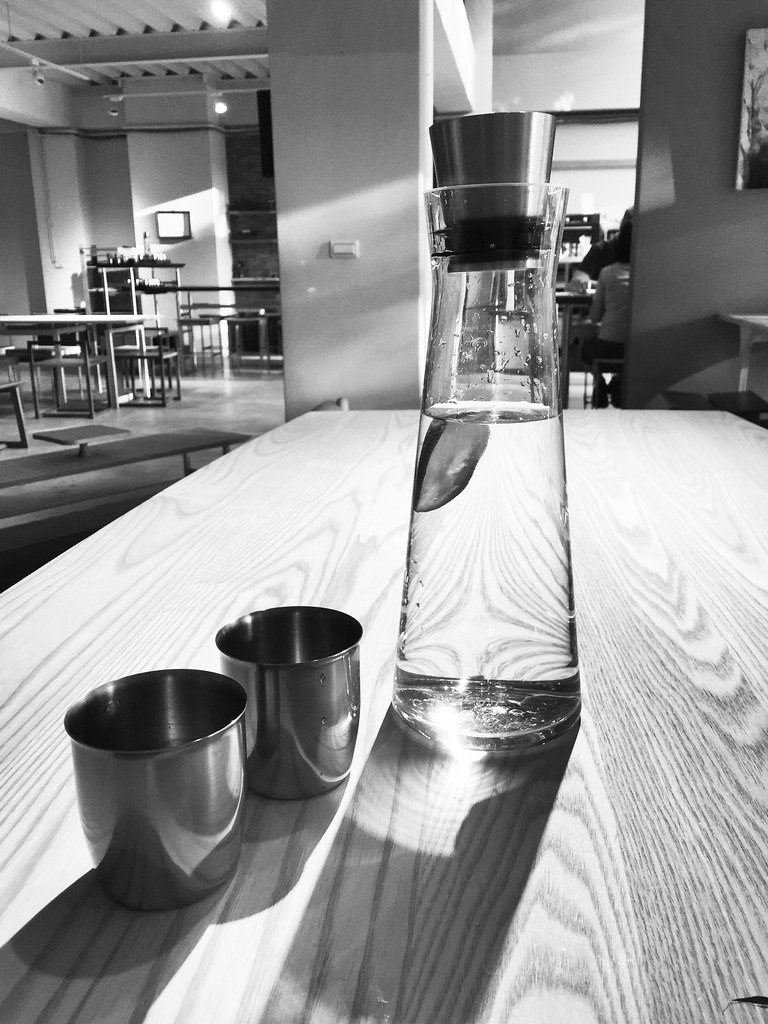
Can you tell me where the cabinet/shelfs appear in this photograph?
[225,207,281,290]
[85,244,190,378]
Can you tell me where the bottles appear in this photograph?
[390,108,583,753]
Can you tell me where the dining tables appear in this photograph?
[0,409,767,1024]
[166,284,283,372]
[554,289,596,409]
[0,313,163,410]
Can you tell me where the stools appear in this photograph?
[6,330,84,404]
[582,359,622,407]
[0,381,31,450]
[555,213,601,293]
[0,345,26,398]
[113,329,182,409]
[26,341,108,419]
[178,304,222,362]
[708,390,768,428]
[225,309,274,371]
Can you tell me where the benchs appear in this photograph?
[0,428,252,553]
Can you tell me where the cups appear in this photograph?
[214,604,363,801]
[63,668,251,913]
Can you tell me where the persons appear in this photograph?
[579,219,633,408]
[571,206,635,290]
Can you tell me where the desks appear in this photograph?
[716,311,768,396]
